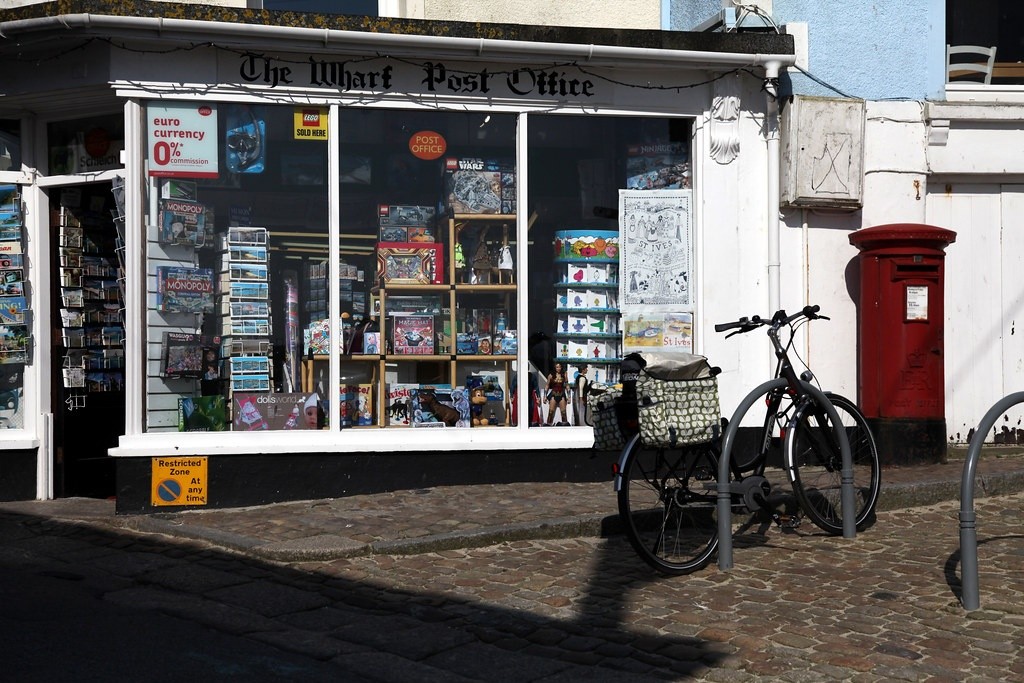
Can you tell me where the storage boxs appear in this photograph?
[158,150,519,428]
[627,130,692,189]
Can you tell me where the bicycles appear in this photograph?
[609,302,884,577]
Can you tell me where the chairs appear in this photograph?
[945,43,997,86]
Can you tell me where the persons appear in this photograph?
[495,312,508,334]
[510,362,541,425]
[292,403,300,428]
[542,362,571,427]
[304,393,320,429]
[574,364,589,426]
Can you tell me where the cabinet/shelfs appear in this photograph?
[213,229,276,393]
[58,185,127,411]
[551,230,621,392]
[303,207,518,427]
[0,181,32,365]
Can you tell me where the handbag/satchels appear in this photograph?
[624,350,723,446]
[587,380,625,450]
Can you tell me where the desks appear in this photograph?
[949,62,1024,84]
